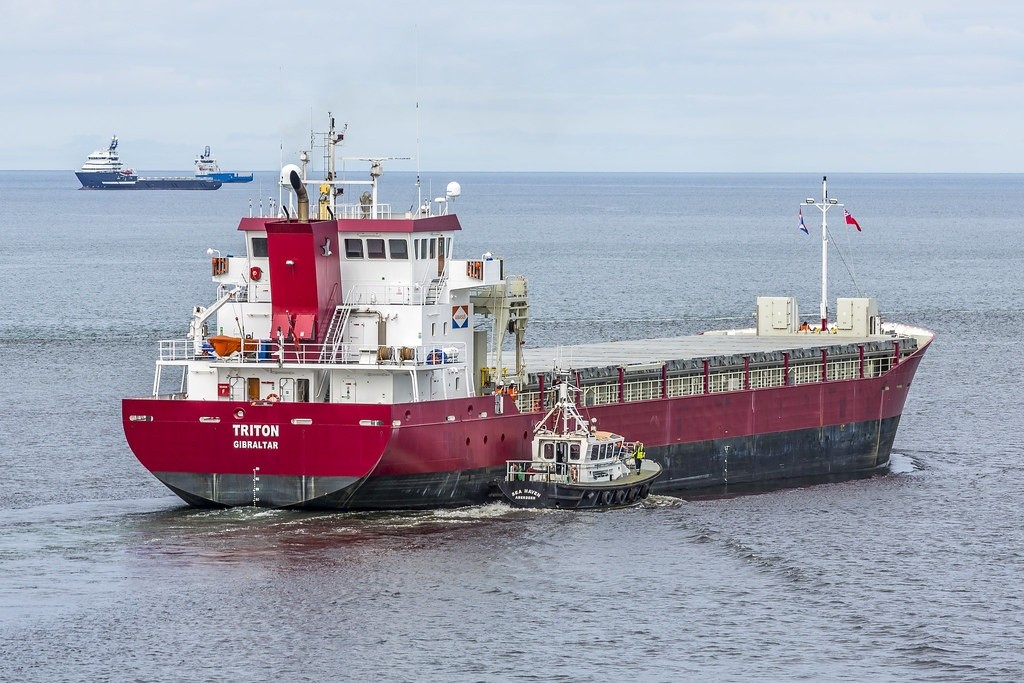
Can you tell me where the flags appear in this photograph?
[797,208,810,235]
[844,209,863,232]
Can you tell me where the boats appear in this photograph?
[117,109,936,511]
[193,145,254,183]
[493,341,663,510]
[74,135,223,190]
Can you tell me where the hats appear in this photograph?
[511,380,515,383]
[500,381,504,385]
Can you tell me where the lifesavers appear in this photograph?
[629,488,638,501]
[602,491,611,505]
[251,267,261,281]
[616,490,625,503]
[266,394,280,403]
[571,467,578,481]
[468,263,481,278]
[641,487,649,499]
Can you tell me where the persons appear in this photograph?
[630,440,646,475]
[800,322,812,331]
[552,376,562,403]
[496,381,506,396]
[508,380,518,401]
